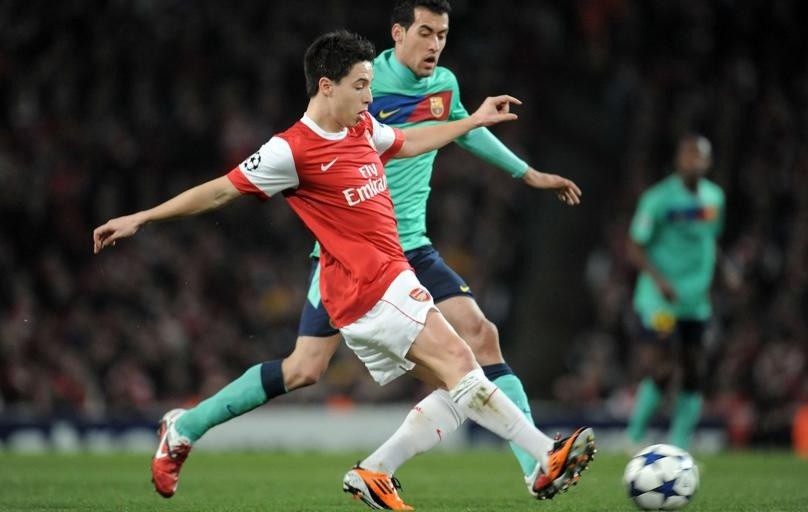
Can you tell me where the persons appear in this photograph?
[621,133,748,457]
[89,29,599,511]
[147,0,585,502]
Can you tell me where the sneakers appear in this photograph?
[343,461,415,512]
[151,409,191,497]
[524,425,597,500]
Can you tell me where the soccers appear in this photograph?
[625,444,700,511]
